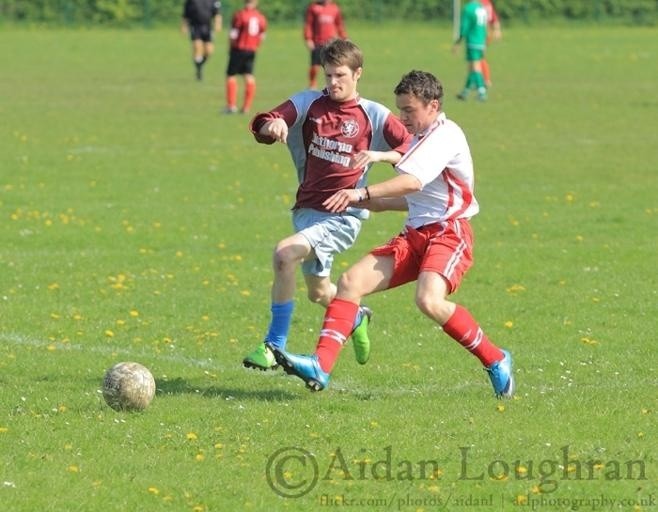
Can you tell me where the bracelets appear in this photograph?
[365,187,370,200]
[356,188,363,202]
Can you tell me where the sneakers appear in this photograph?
[274,349,330,391]
[243,343,280,369]
[484,350,514,400]
[351,307,371,364]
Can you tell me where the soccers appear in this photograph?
[103,362,155,411]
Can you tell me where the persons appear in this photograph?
[304,0,351,90]
[455,0,488,101]
[274,70,514,399]
[222,0,268,114]
[182,0,222,80]
[243,39,414,371]
[478,0,501,87]
